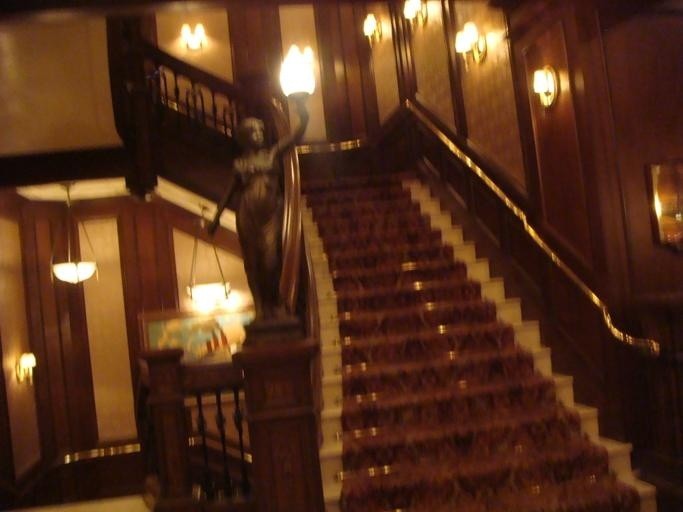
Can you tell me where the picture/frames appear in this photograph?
[140,305,257,368]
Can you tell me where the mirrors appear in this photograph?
[643,159,683,254]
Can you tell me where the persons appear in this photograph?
[206,95,307,322]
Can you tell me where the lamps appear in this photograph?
[15,351,38,386]
[363,13,382,49]
[403,0,428,33]
[533,64,558,111]
[179,1,210,52]
[183,201,253,325]
[48,180,100,284]
[454,20,488,73]
[279,44,316,97]
